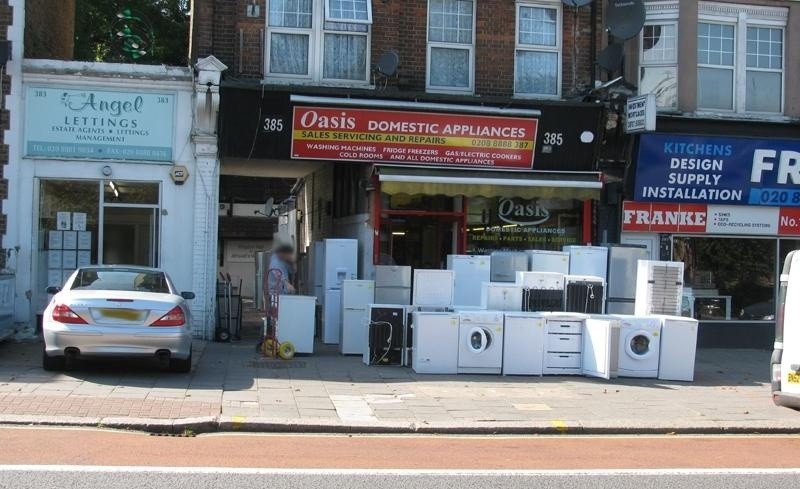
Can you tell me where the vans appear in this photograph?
[769,248,800,416]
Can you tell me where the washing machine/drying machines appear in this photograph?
[459,310,504,374]
[615,313,662,378]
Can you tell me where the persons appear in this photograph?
[257,245,297,350]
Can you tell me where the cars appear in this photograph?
[39,262,195,375]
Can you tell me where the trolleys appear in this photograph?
[256,268,295,361]
[215,270,240,344]
